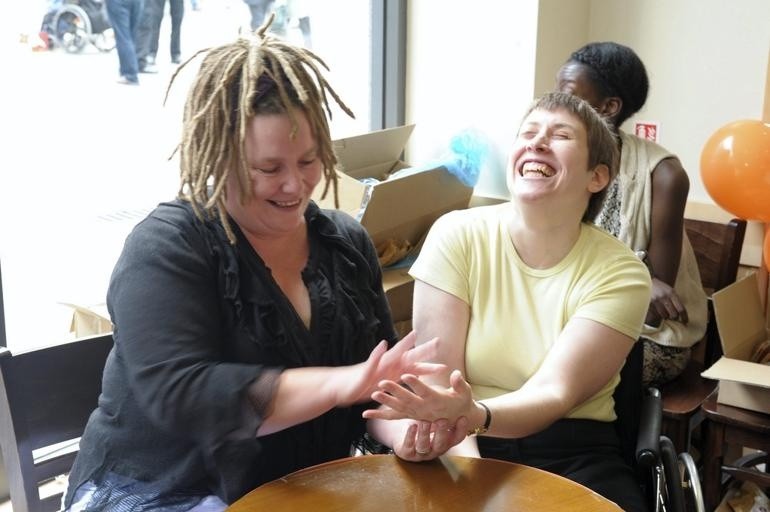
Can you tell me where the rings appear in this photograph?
[416,448,432,456]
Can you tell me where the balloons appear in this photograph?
[700,118,770,273]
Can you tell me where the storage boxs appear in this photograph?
[700,275,769,416]
[380,262,417,322]
[310,125,473,263]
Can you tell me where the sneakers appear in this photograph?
[116,57,183,87]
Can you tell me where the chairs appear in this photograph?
[692,385,769,512]
[1,336,116,512]
[644,218,748,476]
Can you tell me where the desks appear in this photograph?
[224,454,624,512]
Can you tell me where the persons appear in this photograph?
[555,41,708,387]
[362,91,652,512]
[105,0,184,84]
[64,11,448,512]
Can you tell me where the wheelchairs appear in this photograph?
[35,1,116,54]
[612,335,707,512]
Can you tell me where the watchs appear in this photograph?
[466,401,491,437]
